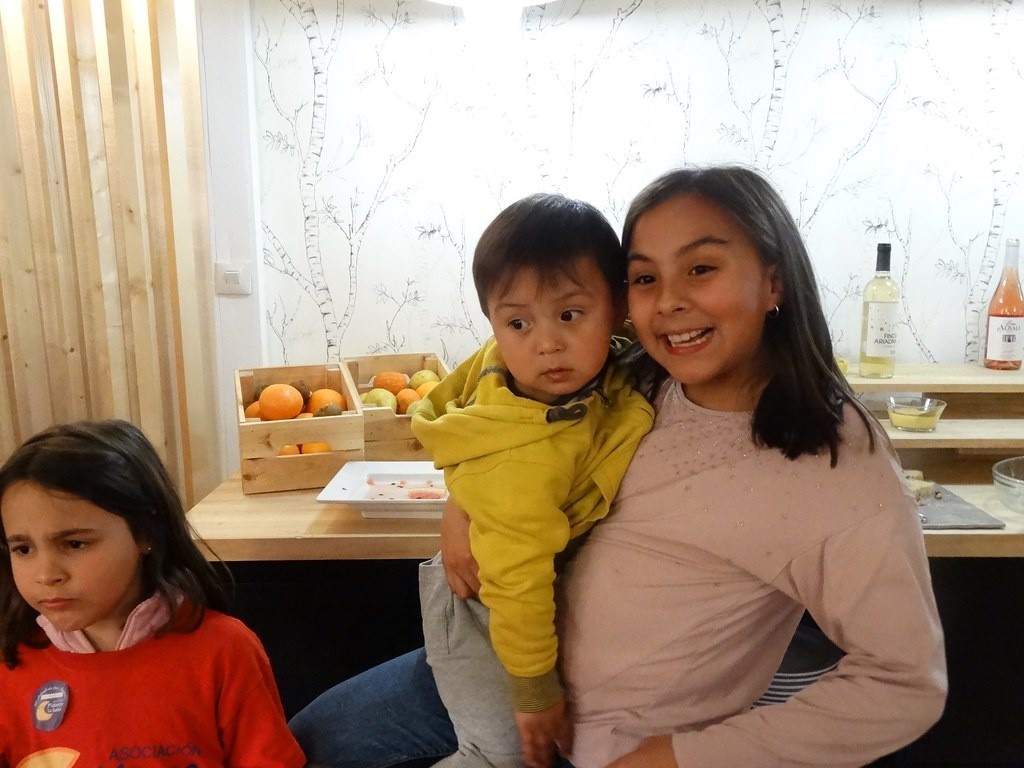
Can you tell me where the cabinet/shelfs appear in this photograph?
[839,363,1024,452]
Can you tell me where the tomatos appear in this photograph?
[243,383,347,456]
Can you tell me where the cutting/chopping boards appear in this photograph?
[917,479,1006,528]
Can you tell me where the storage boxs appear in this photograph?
[234,362,364,495]
[339,349,450,461]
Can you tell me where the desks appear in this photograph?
[184,463,1024,561]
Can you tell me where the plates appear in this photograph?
[317,461,448,519]
[750,625,843,709]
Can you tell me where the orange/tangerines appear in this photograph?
[395,388,423,415]
[372,371,407,394]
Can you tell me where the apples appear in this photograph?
[360,369,444,415]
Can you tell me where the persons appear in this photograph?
[285,166,947,768]
[0,418,309,768]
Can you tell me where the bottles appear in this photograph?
[984,238,1024,370]
[859,243,900,378]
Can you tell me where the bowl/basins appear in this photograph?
[885,397,947,431]
[992,457,1024,515]
